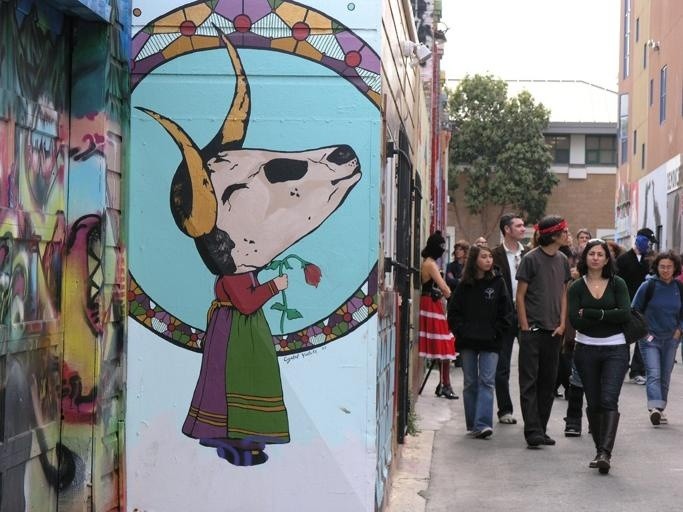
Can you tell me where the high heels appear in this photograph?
[435,383,460,399]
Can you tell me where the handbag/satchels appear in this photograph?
[618,308,649,345]
[431,269,445,302]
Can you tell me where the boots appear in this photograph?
[586,407,602,469]
[563,383,584,437]
[599,410,620,473]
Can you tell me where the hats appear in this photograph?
[636,226,660,244]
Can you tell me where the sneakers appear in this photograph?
[525,432,543,447]
[629,374,647,385]
[471,425,493,439]
[659,413,667,425]
[498,414,518,425]
[541,434,557,444]
[649,407,660,425]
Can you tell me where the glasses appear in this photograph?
[557,228,570,234]
[585,237,607,246]
